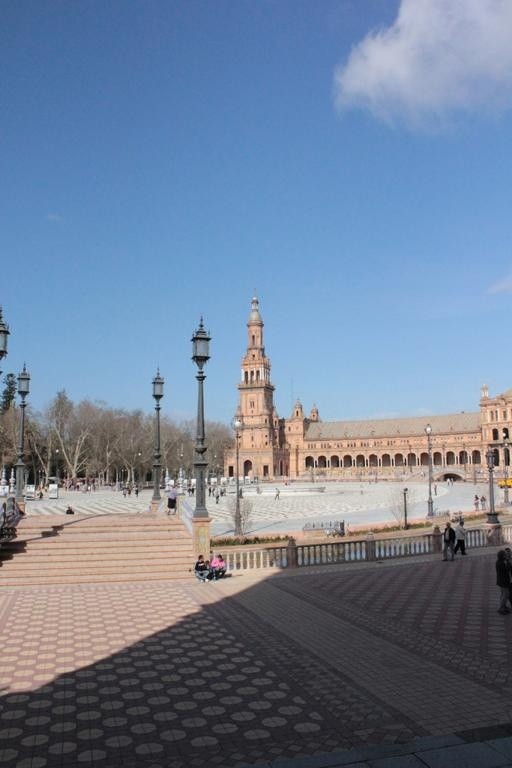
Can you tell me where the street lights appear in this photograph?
[55,448,60,481]
[406,440,478,485]
[230,416,244,537]
[191,313,213,517]
[402,487,410,528]
[12,360,31,499]
[486,444,501,523]
[150,364,166,500]
[0,304,12,363]
[502,472,510,502]
[424,422,436,516]
[503,440,510,473]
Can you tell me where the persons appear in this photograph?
[505,548,511,608]
[441,522,456,562]
[63,476,289,505]
[208,554,227,580]
[473,494,480,511]
[479,495,486,510]
[452,520,468,556]
[66,506,75,514]
[496,549,511,614]
[205,561,213,580]
[166,485,178,516]
[194,554,211,583]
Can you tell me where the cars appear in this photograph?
[324,528,344,538]
[497,478,512,488]
